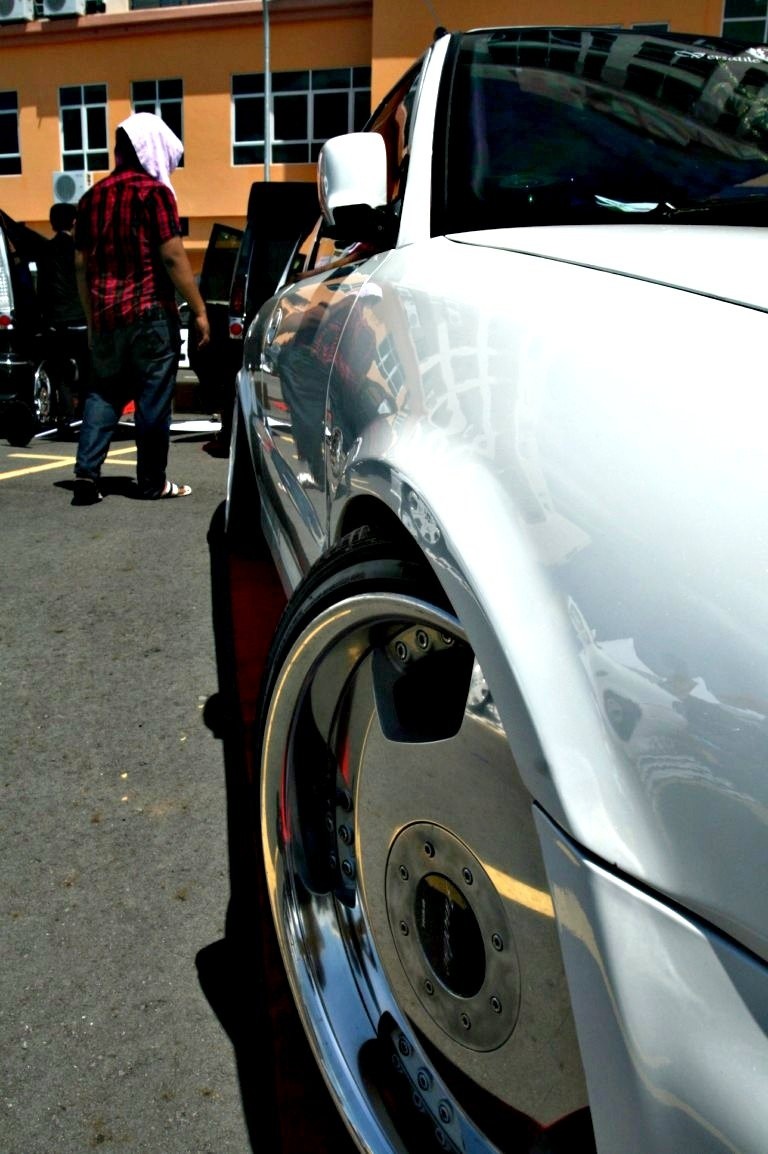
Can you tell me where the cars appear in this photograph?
[178,175,319,422]
[0,209,79,450]
[222,16,767,1152]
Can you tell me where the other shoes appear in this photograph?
[157,481,191,498]
[74,477,103,501]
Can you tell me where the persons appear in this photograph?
[72,112,209,502]
[270,282,383,492]
[49,203,76,251]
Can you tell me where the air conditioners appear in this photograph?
[0,0,34,22]
[53,170,94,205]
[42,0,86,17]
